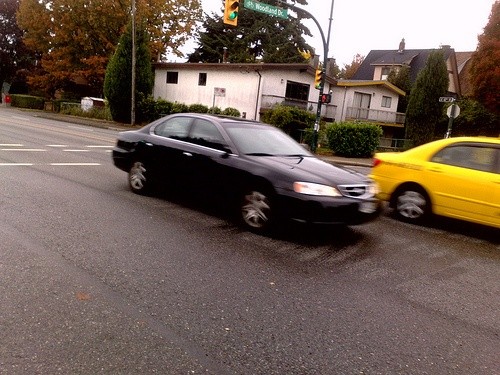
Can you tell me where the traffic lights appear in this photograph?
[224,0,239,26]
[315,70,323,90]
[322,94,332,104]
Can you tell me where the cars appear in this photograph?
[111,113,384,233]
[369,137,500,228]
